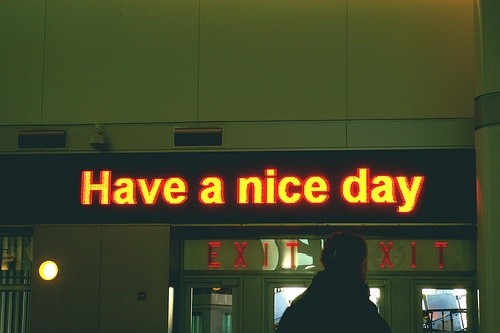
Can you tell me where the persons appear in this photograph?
[275,228,393,333]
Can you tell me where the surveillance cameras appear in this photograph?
[89,128,105,149]
